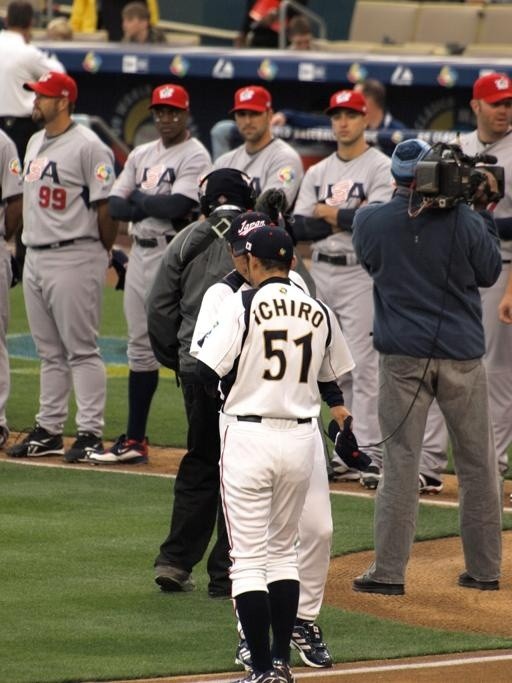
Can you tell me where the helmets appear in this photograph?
[197,167,256,216]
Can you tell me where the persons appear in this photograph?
[236,0,307,48]
[86,85,214,466]
[414,72,512,497]
[0,0,67,172]
[287,15,315,51]
[269,79,407,156]
[0,126,23,452]
[68,1,159,34]
[44,16,72,41]
[122,3,167,43]
[212,86,304,214]
[351,138,503,595]
[292,89,394,491]
[4,72,118,465]
[144,168,380,681]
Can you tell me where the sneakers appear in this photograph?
[84,434,151,465]
[5,422,66,457]
[62,429,106,463]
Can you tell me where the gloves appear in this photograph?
[323,415,372,474]
[113,245,128,289]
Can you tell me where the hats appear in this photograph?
[389,138,434,188]
[228,84,273,113]
[148,83,191,112]
[23,70,78,103]
[224,210,293,263]
[324,89,367,115]
[472,72,512,104]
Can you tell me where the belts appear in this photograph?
[235,414,314,424]
[32,239,74,250]
[317,252,347,266]
[136,234,174,247]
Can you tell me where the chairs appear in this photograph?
[348,0,512,58]
